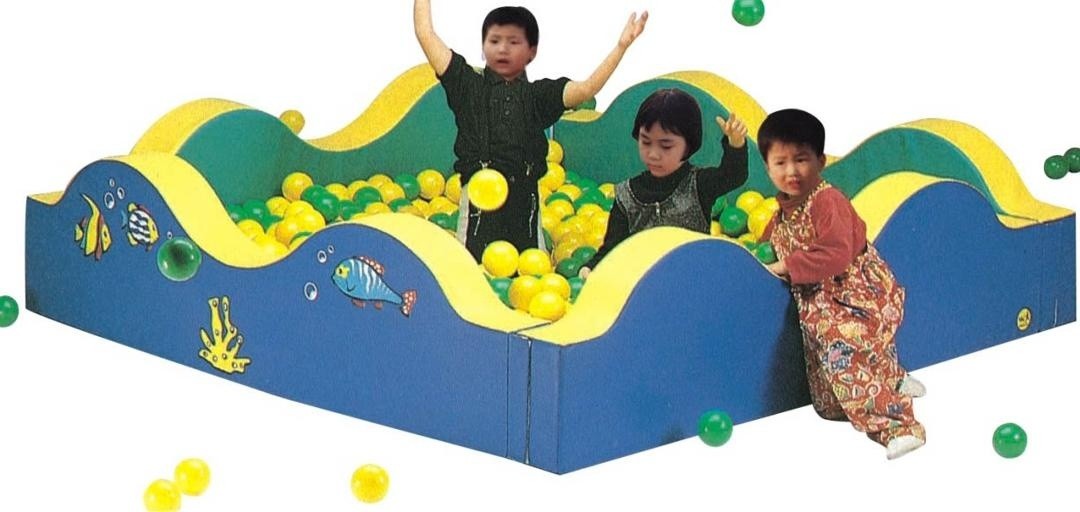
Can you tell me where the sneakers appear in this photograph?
[885,428,926,461]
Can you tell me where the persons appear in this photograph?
[412,0,649,278]
[755,109,927,460]
[578,87,748,279]
